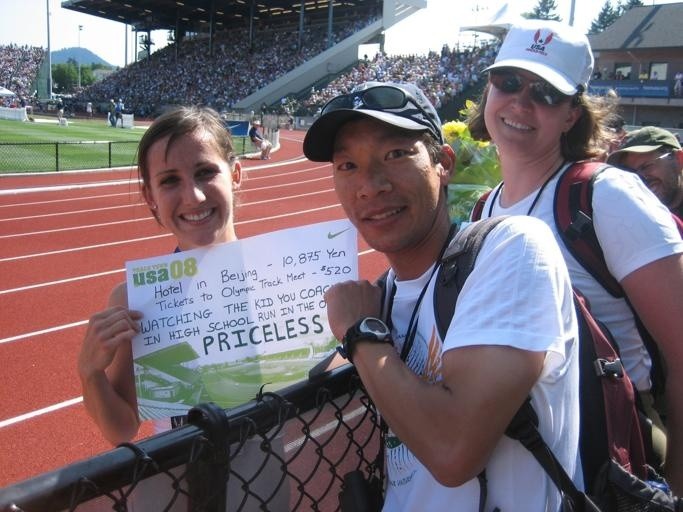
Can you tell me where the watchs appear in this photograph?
[335,316,395,362]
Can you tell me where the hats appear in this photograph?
[607,125,681,164]
[482,18,595,96]
[303,81,444,163]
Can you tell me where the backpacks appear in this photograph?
[433,216,676,511]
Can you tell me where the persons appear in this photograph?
[249,120,272,160]
[0,41,47,122]
[594,67,682,97]
[75,103,294,509]
[468,18,683,511]
[303,82,585,512]
[379,29,507,112]
[48,13,381,130]
[608,125,683,220]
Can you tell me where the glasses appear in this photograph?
[632,153,672,173]
[488,71,562,109]
[319,85,446,146]
